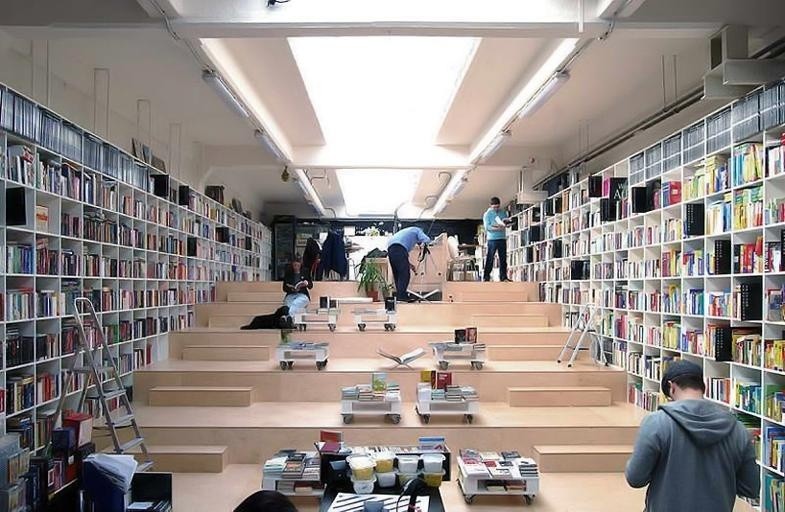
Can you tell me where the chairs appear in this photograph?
[305,237,322,279]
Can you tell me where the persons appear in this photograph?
[623,358,760,512]
[386,223,430,302]
[484,196,513,283]
[277,257,314,330]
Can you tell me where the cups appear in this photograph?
[345,449,446,494]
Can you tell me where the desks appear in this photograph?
[431,342,487,371]
[345,243,363,280]
[261,445,541,512]
[350,310,398,332]
[447,243,477,281]
[278,341,330,371]
[407,245,446,284]
[340,384,402,424]
[292,307,340,332]
[415,386,477,426]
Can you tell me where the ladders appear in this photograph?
[557,303,609,367]
[41,298,155,474]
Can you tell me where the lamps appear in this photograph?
[477,129,511,164]
[294,179,309,197]
[253,127,282,162]
[518,70,568,120]
[434,177,468,217]
[199,70,251,123]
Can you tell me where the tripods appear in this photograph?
[409,248,453,303]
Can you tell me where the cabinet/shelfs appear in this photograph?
[478,74,785,510]
[321,231,345,282]
[0,82,279,506]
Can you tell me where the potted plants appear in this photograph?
[353,259,389,302]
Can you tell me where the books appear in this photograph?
[339,367,479,402]
[262,430,540,512]
[286,281,304,292]
[569,173,628,369]
[703,134,785,511]
[492,215,510,227]
[275,340,329,350]
[376,345,425,364]
[429,327,486,352]
[507,190,570,328]
[628,163,703,410]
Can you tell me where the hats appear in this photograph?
[661,357,705,395]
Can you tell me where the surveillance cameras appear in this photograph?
[281,172,289,182]
